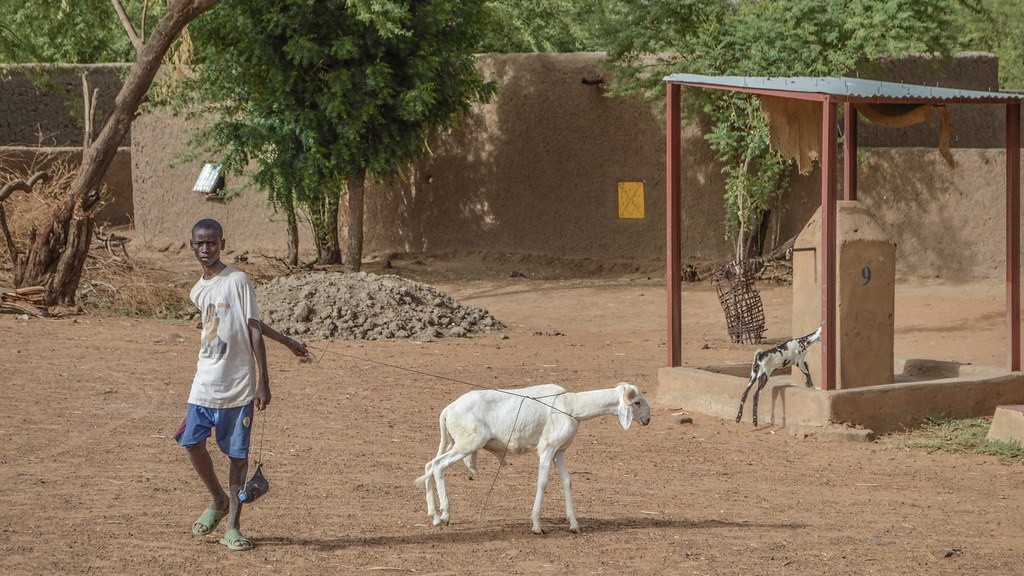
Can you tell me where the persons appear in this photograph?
[175,219,308,550]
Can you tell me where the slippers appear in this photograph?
[192,506,229,535]
[219,529,253,551]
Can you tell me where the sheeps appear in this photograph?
[412,382,651,535]
[735,326,822,428]
